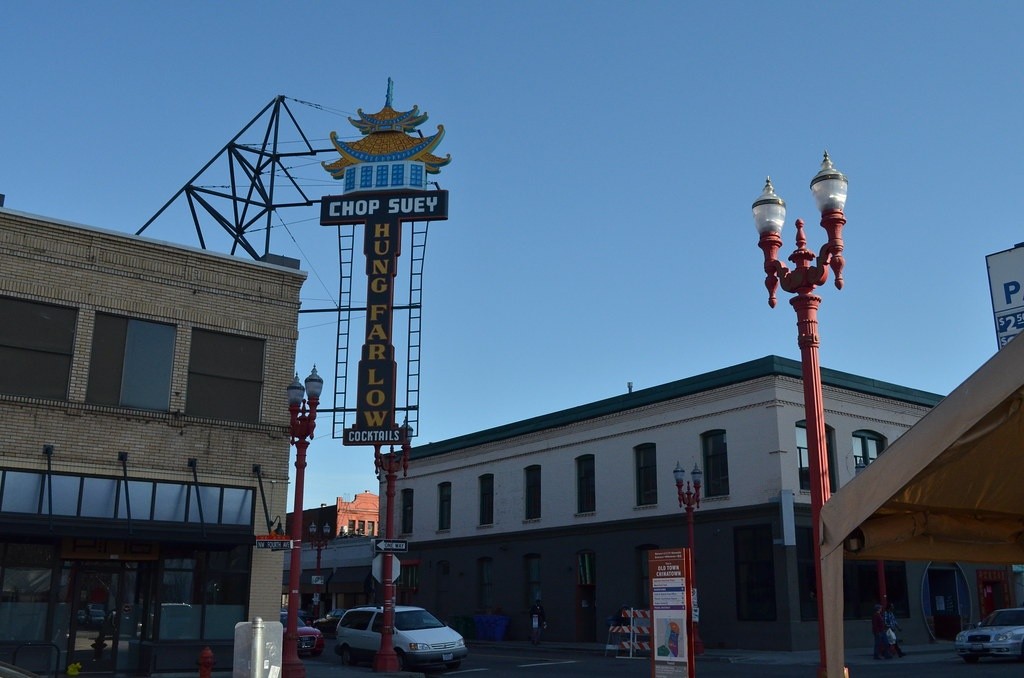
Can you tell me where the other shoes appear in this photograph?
[874,655,883,659]
[899,653,906,658]
[885,654,894,659]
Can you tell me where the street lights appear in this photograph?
[281,362,323,678]
[308,520,332,619]
[673,459,706,655]
[751,148,848,678]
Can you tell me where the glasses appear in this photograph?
[890,606,894,608]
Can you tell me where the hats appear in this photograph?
[874,605,883,610]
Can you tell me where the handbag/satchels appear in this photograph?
[886,628,896,645]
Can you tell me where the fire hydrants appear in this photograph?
[198,645,219,678]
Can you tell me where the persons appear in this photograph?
[872,602,907,660]
[529,600,545,645]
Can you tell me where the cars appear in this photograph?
[78,603,105,630]
[955,607,1024,664]
[281,611,324,658]
[312,607,349,636]
[334,604,469,673]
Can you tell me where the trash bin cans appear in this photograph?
[473,614,511,642]
